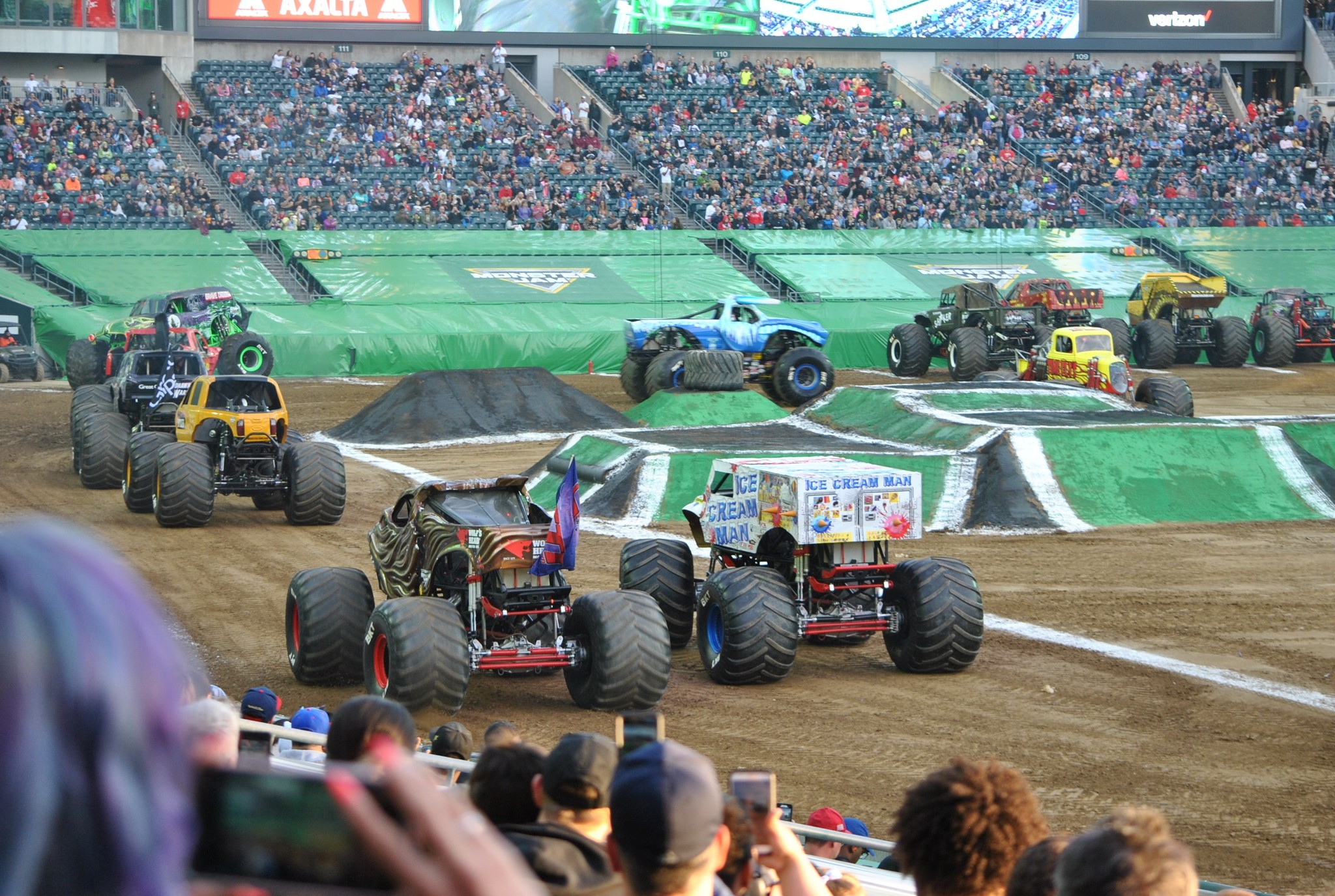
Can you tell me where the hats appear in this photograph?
[612,740,722,865]
[807,807,853,835]
[430,721,473,760]
[542,731,619,809]
[842,817,876,856]
[240,687,282,719]
[292,707,329,734]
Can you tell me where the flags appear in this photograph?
[528,455,580,576]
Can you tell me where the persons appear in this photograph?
[0,42,1335,230]
[0,330,17,348]
[0,509,1255,896]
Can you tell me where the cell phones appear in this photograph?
[615,712,665,749]
[729,770,776,853]
[191,770,410,896]
[777,803,793,822]
[238,731,272,774]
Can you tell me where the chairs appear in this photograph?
[0,60,1335,230]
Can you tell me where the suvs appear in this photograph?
[978,324,1204,413]
[278,475,699,706]
[1251,287,1335,368]
[1125,272,1254,366]
[123,371,347,527]
[618,460,986,685]
[6,322,43,380]
[886,282,1064,378]
[620,295,836,414]
[1000,278,1138,368]
[63,285,275,488]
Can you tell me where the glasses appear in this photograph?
[858,852,869,859]
